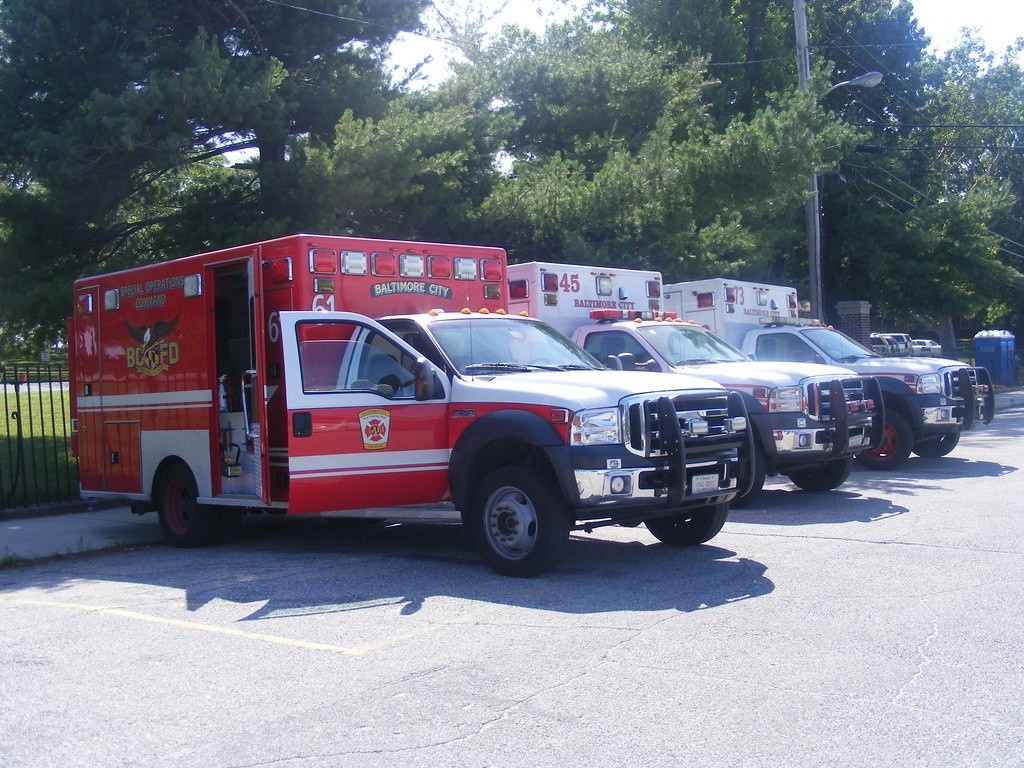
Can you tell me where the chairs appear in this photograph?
[368,355,403,392]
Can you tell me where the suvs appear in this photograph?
[869,332,914,358]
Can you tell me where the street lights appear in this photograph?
[807,71,885,321]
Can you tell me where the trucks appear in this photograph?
[71,232,755,578]
[505,262,885,509]
[660,278,996,471]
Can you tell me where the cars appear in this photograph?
[912,339,942,358]
